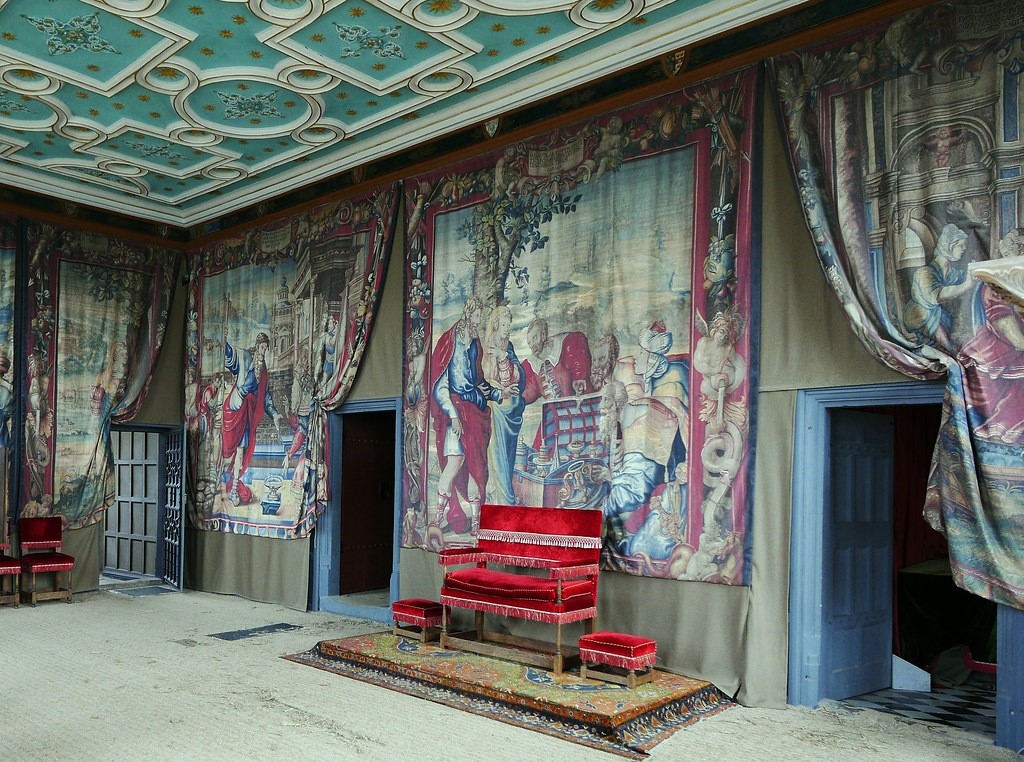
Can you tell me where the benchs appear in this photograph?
[439,506,603,676]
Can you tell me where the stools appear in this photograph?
[577,630,657,688]
[391,598,452,644]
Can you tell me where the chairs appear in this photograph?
[0,543,22,608]
[17,515,76,607]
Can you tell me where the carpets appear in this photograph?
[115,587,177,597]
[279,624,738,762]
